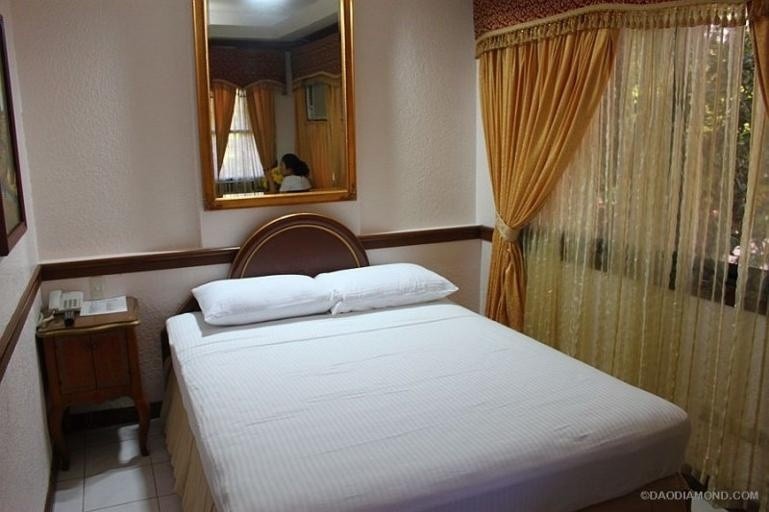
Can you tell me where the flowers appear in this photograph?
[260,166,284,190]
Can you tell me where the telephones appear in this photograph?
[48,289,83,314]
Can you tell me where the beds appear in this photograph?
[159,209,693,512]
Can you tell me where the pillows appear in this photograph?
[189,272,344,327]
[314,262,461,316]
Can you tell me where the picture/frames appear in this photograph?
[0,15,28,256]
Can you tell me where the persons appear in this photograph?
[263,152,311,194]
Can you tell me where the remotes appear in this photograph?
[63,310,76,324]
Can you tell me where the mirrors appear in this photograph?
[192,0,358,211]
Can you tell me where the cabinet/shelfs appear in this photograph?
[36,296,152,473]
